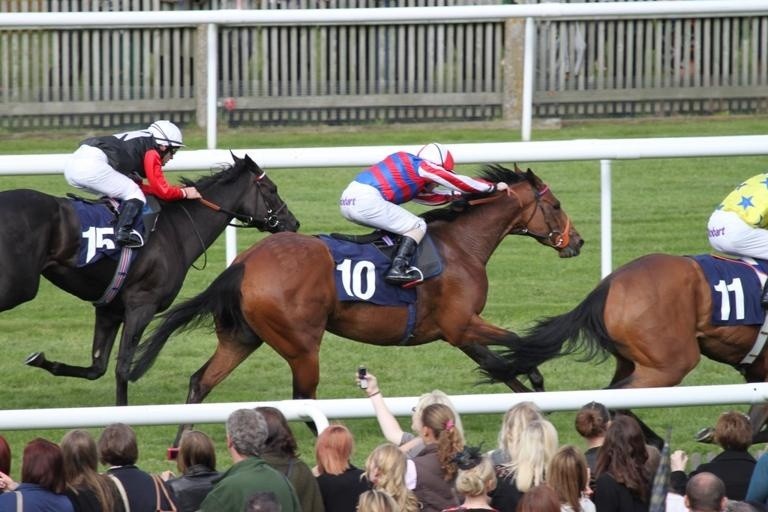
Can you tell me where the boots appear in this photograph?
[761,277,768,310]
[114,198,140,246]
[384,237,420,286]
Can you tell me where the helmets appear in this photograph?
[147,120,186,147]
[418,142,457,175]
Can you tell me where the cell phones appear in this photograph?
[358,367,368,389]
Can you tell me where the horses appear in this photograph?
[118,159,585,459]
[452,251,768,453]
[0,149,302,408]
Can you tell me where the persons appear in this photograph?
[64,120,203,247]
[707,172,768,307]
[1,365,767,512]
[340,142,510,285]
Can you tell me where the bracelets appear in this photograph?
[181,187,187,200]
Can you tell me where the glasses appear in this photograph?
[149,123,177,154]
